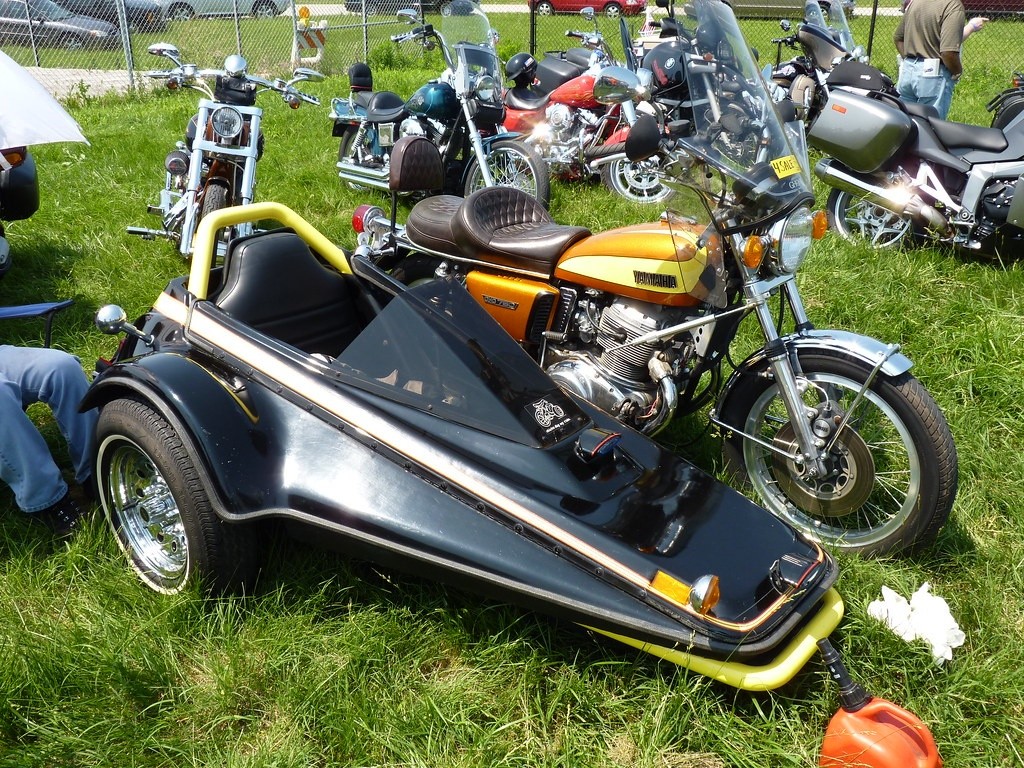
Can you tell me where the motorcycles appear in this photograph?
[330,0,958,560]
[125,41,327,262]
[806,61,1024,265]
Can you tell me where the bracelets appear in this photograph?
[954,71,963,78]
[970,22,978,32]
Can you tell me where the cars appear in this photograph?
[0,0,120,51]
[529,0,646,18]
[160,0,291,22]
[684,0,856,19]
[62,0,165,33]
[344,0,479,16]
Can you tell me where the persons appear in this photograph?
[0,345,104,534]
[893,0,989,119]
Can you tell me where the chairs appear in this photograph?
[346,61,378,108]
[224,217,381,366]
[0,298,76,411]
[387,134,465,249]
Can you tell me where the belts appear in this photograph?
[907,54,924,61]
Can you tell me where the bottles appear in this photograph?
[817,638,942,768]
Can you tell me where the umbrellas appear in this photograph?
[0,50,91,151]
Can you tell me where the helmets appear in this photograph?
[505,53,538,82]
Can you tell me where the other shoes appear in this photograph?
[45,495,90,538]
